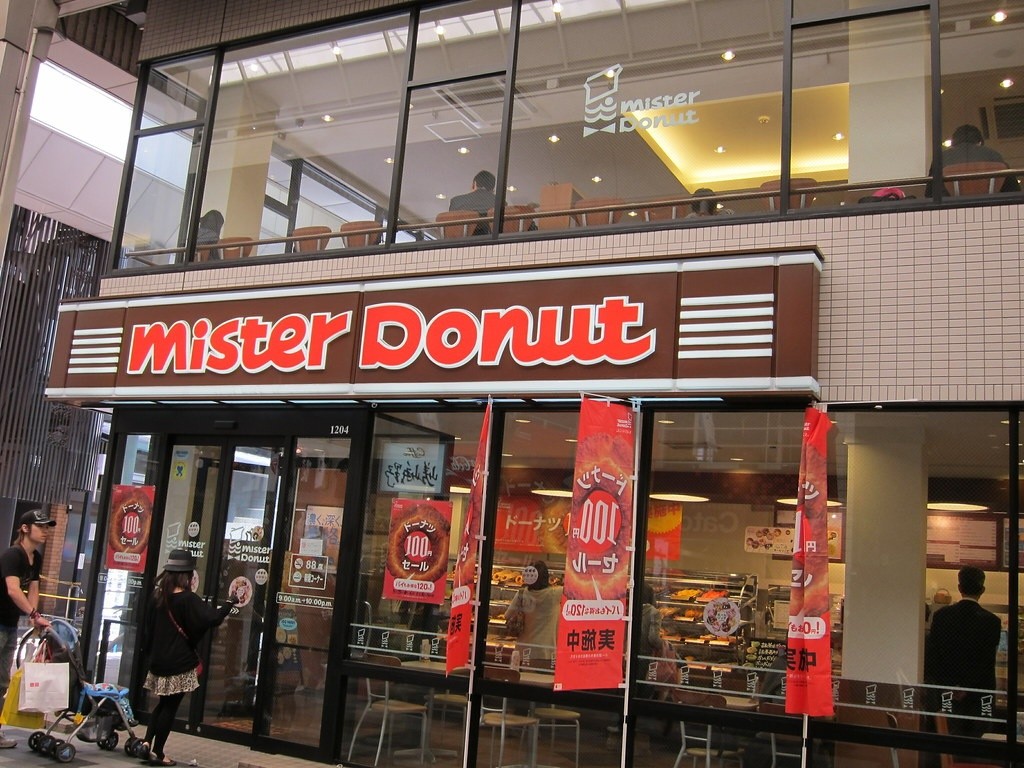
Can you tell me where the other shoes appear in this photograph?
[149,754,177,766]
[137,745,149,760]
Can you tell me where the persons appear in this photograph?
[691,189,734,217]
[197,209,224,260]
[139,550,239,765]
[398,601,439,652]
[636,583,672,682]
[759,654,787,705]
[926,124,1022,198]
[0,508,58,747]
[927,566,1001,738]
[449,171,508,236]
[504,561,564,670]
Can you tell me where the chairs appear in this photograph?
[292,222,381,253]
[887,711,913,768]
[941,162,1007,198]
[670,686,745,768]
[346,655,471,768]
[761,177,818,213]
[185,236,257,264]
[758,702,816,768]
[936,714,1001,768]
[476,666,583,768]
[573,196,686,228]
[435,204,534,239]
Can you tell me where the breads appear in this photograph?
[656,589,743,643]
[497,613,505,619]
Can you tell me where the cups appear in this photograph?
[420,644,430,663]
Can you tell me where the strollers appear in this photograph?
[28,621,150,763]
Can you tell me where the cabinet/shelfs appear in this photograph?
[407,555,759,681]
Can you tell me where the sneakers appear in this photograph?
[0,733,17,748]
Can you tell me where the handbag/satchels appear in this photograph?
[646,640,683,693]
[507,588,527,637]
[0,639,69,728]
[197,663,202,676]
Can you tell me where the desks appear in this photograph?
[396,660,470,763]
[504,672,557,767]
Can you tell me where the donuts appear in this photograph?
[492,570,560,584]
[386,504,451,585]
[563,431,636,603]
[108,490,154,554]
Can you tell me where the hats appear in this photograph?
[163,550,198,571]
[18,509,57,527]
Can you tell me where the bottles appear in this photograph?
[511,651,520,672]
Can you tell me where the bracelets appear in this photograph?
[29,608,38,620]
